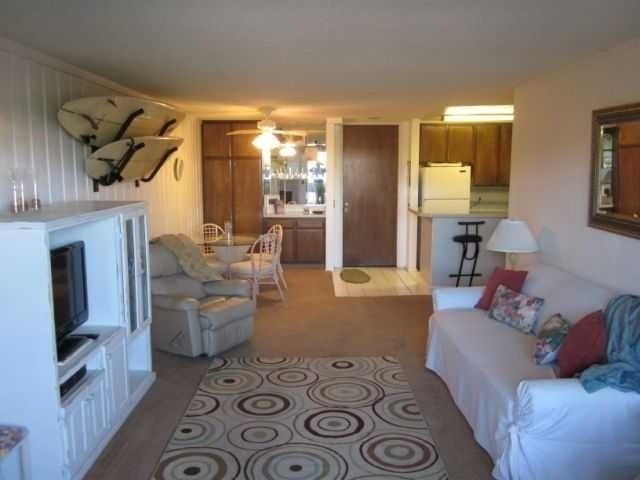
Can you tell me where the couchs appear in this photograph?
[423,258,640,480]
[149,232,258,358]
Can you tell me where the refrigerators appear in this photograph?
[419,164,472,215]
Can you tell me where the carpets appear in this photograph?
[332,267,430,298]
[147,355,450,480]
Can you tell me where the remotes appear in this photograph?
[70,333,99,341]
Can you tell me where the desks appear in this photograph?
[195,236,257,278]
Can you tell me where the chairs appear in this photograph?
[250,224,288,288]
[229,233,285,307]
[448,220,485,286]
[197,223,225,259]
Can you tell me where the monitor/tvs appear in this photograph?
[51,239,94,368]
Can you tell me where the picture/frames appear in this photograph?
[175,158,184,181]
[586,102,640,241]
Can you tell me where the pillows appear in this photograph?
[473,264,528,312]
[488,283,545,335]
[534,313,569,366]
[558,309,608,379]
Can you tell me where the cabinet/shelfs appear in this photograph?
[0,199,156,480]
[202,119,262,238]
[263,216,326,264]
[475,124,512,187]
[420,123,475,186]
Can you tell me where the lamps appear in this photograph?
[486,218,539,270]
[225,107,306,152]
